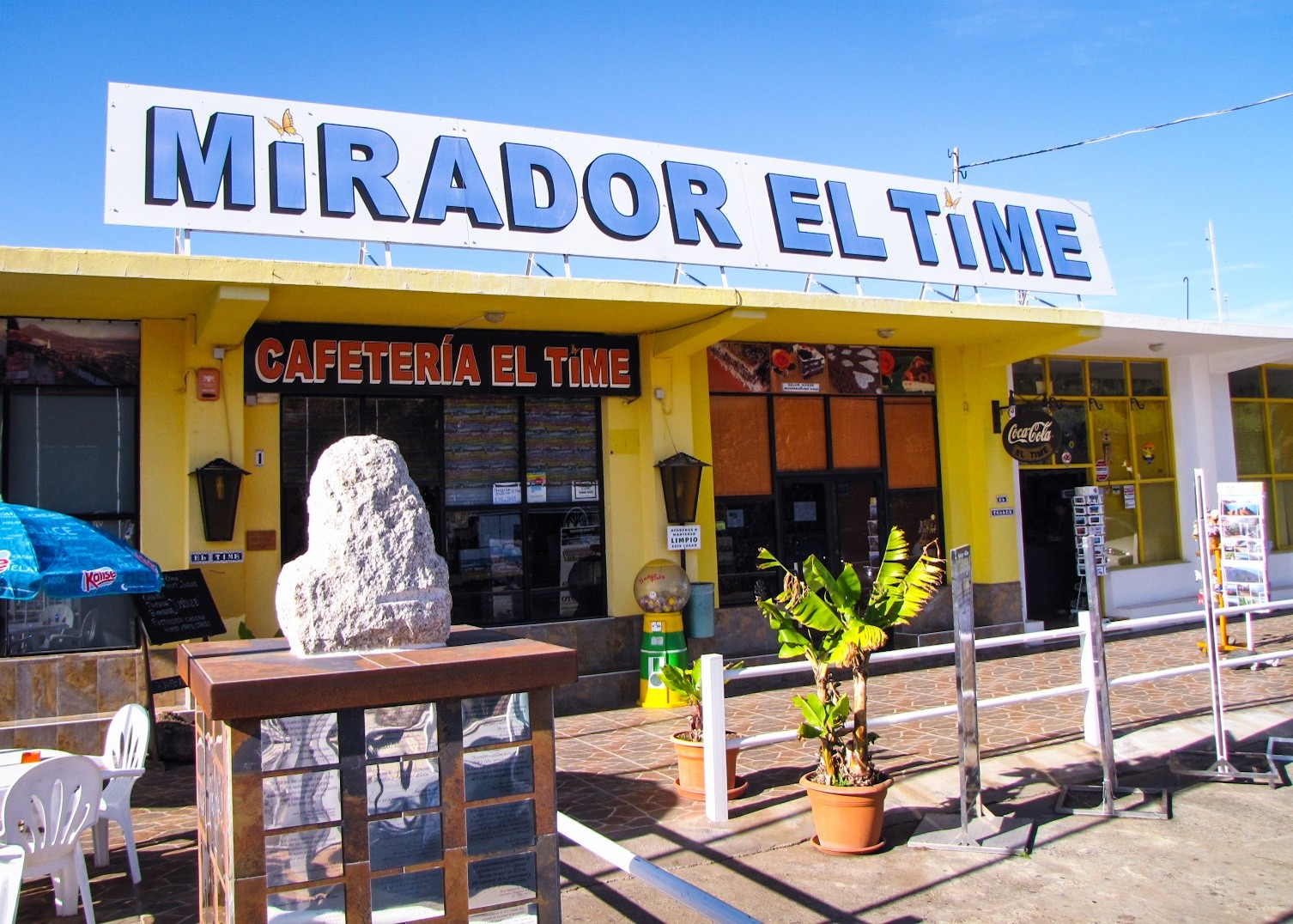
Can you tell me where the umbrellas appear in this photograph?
[0,494,164,601]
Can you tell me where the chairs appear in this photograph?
[49,608,99,651]
[80,703,151,886]
[36,603,74,652]
[0,755,105,924]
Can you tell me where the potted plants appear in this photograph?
[659,657,749,801]
[754,525,947,857]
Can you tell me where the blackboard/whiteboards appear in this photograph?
[132,566,227,646]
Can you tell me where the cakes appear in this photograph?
[708,342,936,391]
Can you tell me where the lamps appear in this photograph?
[189,455,251,542]
[655,451,712,524]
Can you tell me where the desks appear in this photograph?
[0,749,79,916]
[7,621,67,652]
